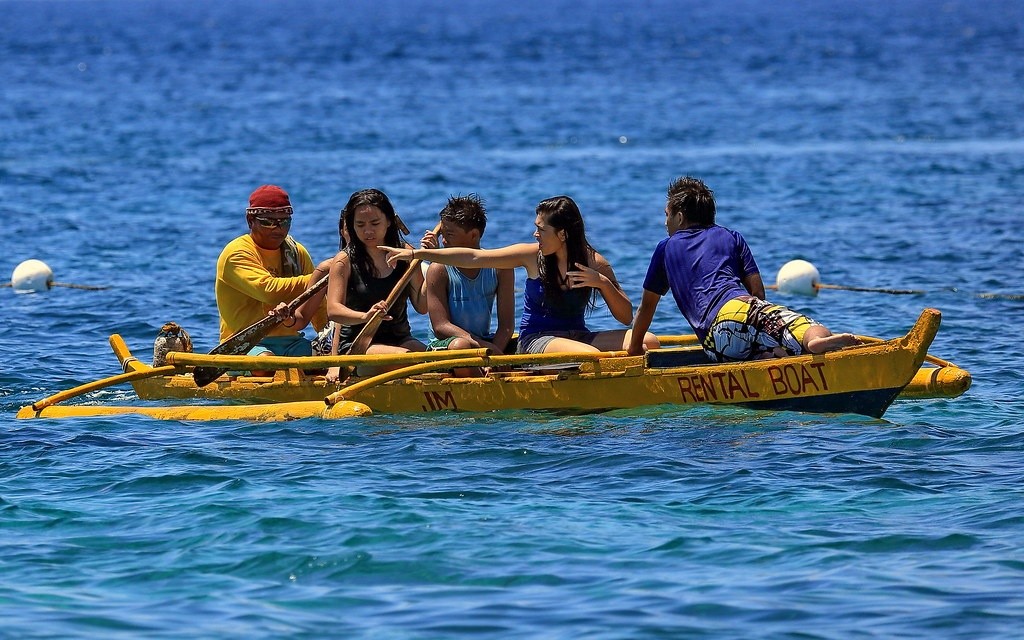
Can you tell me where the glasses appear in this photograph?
[254,216,292,229]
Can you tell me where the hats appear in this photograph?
[246,184,293,215]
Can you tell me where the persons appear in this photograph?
[215,181,348,382]
[326,187,440,379]
[628,179,862,356]
[376,197,660,376]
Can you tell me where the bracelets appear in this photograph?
[410,250,415,258]
[280,314,298,328]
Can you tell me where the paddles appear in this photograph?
[336,213,444,385]
[192,273,326,390]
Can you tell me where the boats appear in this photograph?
[108,308,944,425]
